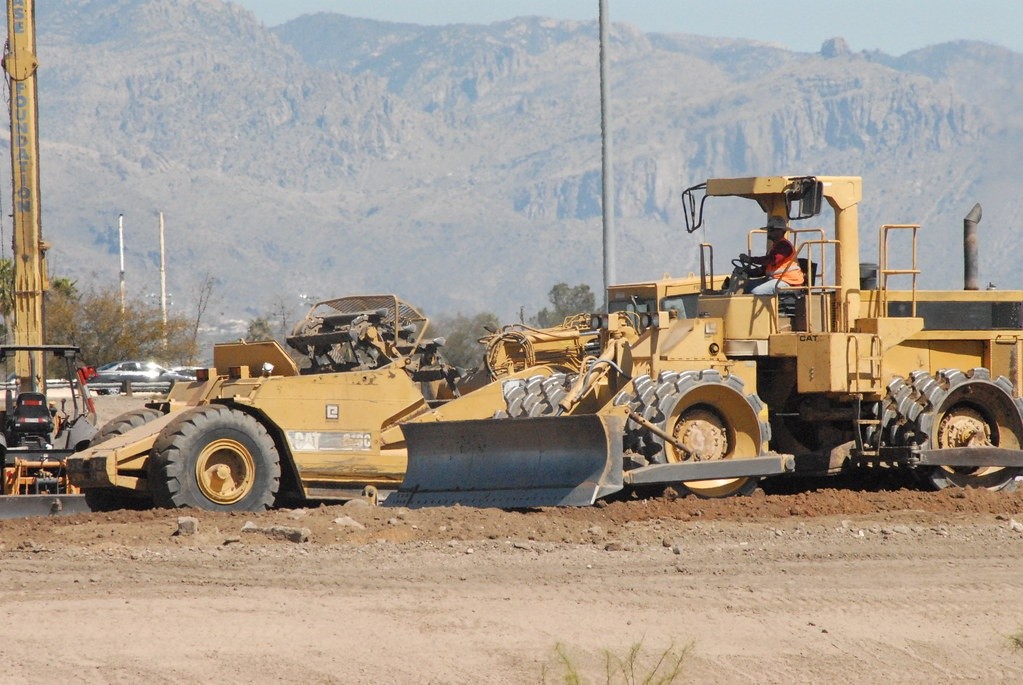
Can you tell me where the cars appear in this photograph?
[74,360,181,395]
[167,366,205,381]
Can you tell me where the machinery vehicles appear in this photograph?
[66,275,733,513]
[0,0,101,519]
[380,175,1023,509]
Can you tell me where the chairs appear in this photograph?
[777,257,817,318]
[10,392,54,434]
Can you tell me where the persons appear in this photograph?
[740,215,804,295]
[669,307,678,319]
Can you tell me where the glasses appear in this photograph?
[767,228,782,233]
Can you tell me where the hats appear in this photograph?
[760,216,794,232]
